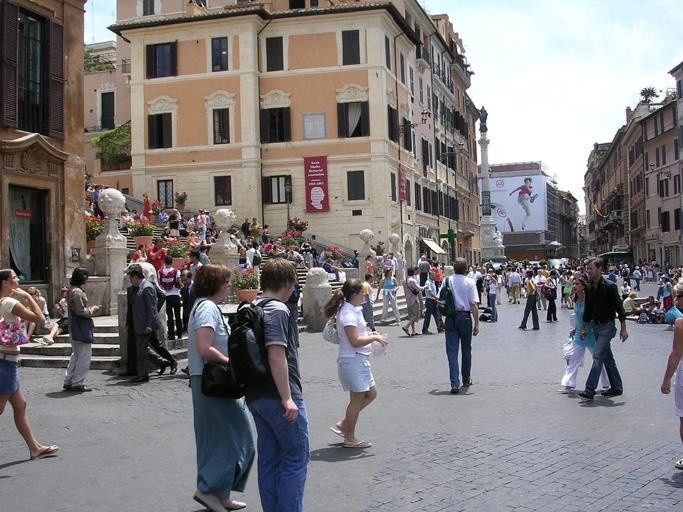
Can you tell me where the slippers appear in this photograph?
[330,424,345,438]
[411,332,422,335]
[30,445,59,460]
[343,439,372,448]
[402,327,410,336]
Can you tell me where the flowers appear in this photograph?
[85,183,345,288]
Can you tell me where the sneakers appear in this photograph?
[422,329,432,334]
[131,375,149,382]
[119,370,136,376]
[601,387,623,396]
[675,459,683,468]
[463,378,472,386]
[71,386,92,392]
[169,361,177,374]
[557,387,576,393]
[451,386,459,393]
[63,384,72,390]
[219,498,246,510]
[579,389,596,399]
[159,361,171,375]
[193,489,228,512]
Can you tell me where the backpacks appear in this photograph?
[439,277,455,317]
[531,279,541,300]
[253,251,261,265]
[225,296,291,388]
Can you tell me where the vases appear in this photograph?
[236,288,259,303]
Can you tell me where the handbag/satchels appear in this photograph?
[412,289,419,295]
[70,314,94,344]
[201,362,244,399]
[322,316,339,343]
[0,297,29,346]
